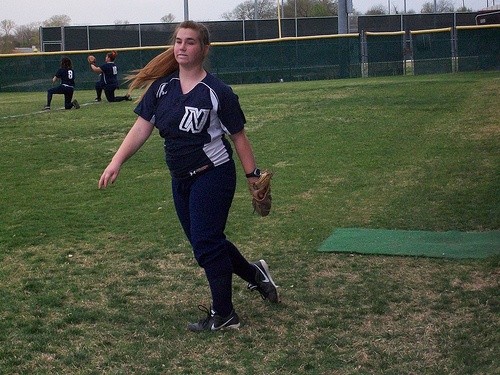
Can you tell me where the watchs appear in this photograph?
[246,168,261,179]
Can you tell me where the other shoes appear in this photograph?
[94,97,101,101]
[125,96,132,101]
[42,106,50,111]
[72,99,80,109]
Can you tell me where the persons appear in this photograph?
[98,20,279,332]
[89,50,131,103]
[42,57,80,110]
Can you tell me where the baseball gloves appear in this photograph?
[88,56,96,64]
[251,169,275,218]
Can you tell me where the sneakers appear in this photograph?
[247,259,280,305]
[186,303,241,333]
